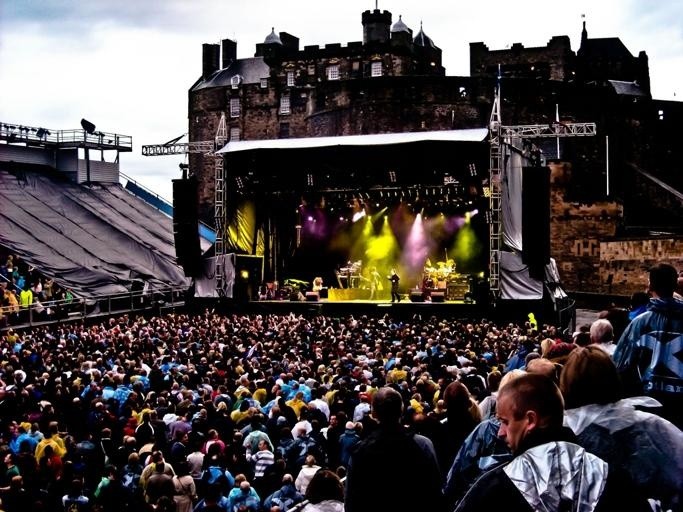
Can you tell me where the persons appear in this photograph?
[0,254,683,512]
